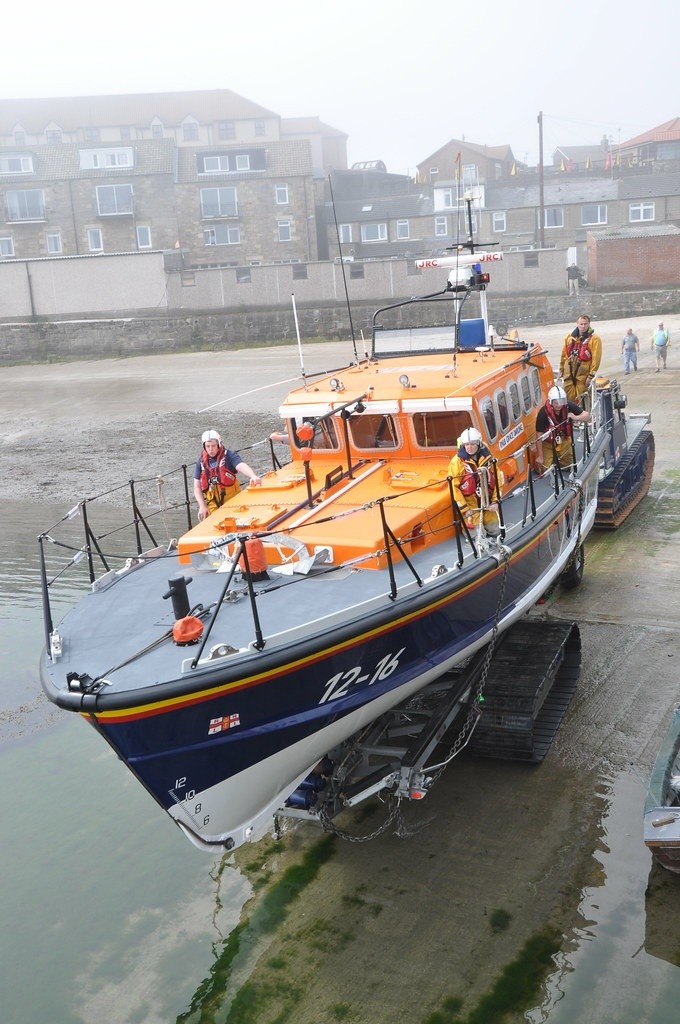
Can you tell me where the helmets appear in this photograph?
[201,429,222,449]
[547,386,568,406]
[460,427,482,447]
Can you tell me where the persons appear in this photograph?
[650,322,671,373]
[556,316,602,406]
[535,385,591,474]
[622,329,640,373]
[193,429,262,522]
[448,427,502,540]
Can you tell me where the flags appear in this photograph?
[534,164,539,173]
[560,157,572,170]
[616,154,623,165]
[586,157,594,168]
[510,164,517,176]
[603,153,610,168]
[454,152,460,162]
[627,157,632,167]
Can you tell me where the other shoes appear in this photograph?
[655,363,666,373]
[624,366,638,375]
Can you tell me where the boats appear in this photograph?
[38,189,632,851]
